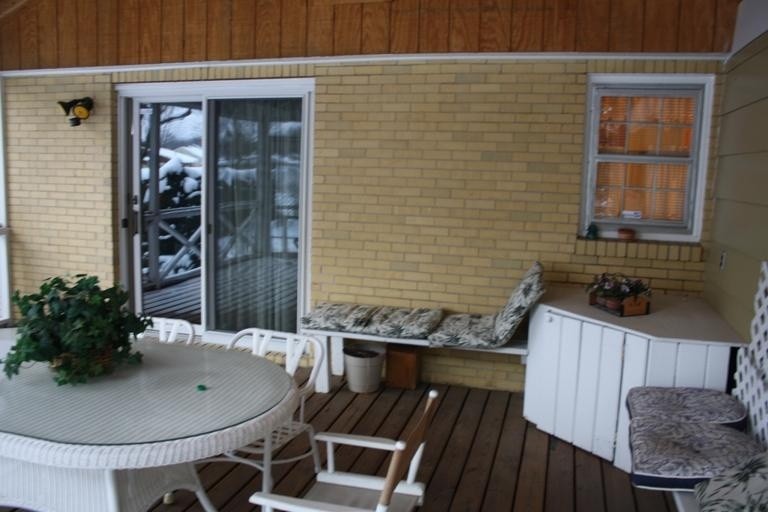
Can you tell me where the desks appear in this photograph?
[0,325,300,512]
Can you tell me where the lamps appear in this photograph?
[55,96,93,128]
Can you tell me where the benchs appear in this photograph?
[301,327,529,393]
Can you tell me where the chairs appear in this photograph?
[247,388,440,512]
[137,316,323,511]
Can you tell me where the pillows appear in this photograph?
[299,261,545,349]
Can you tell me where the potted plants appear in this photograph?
[0,270,156,387]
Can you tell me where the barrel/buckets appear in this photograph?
[342,346,385,394]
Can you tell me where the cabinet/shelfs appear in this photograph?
[522,280,748,476]
